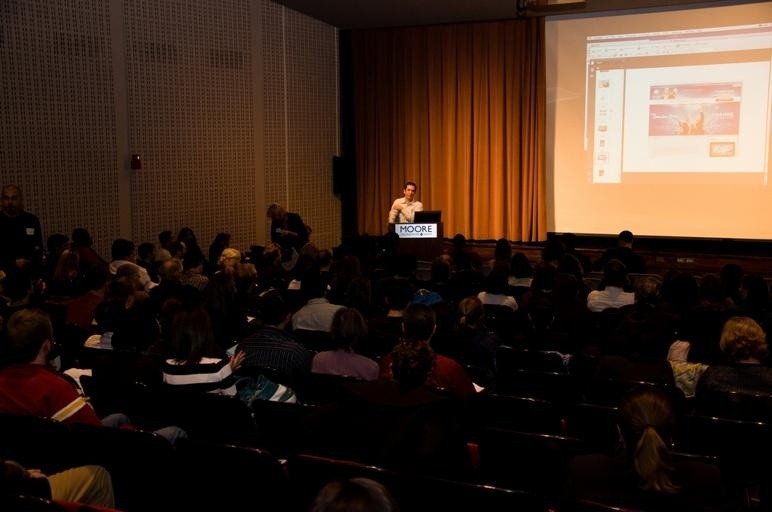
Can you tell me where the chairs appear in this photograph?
[0,286,770,511]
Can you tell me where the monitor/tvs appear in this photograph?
[414,211,441,223]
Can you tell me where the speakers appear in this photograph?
[332,156,357,194]
